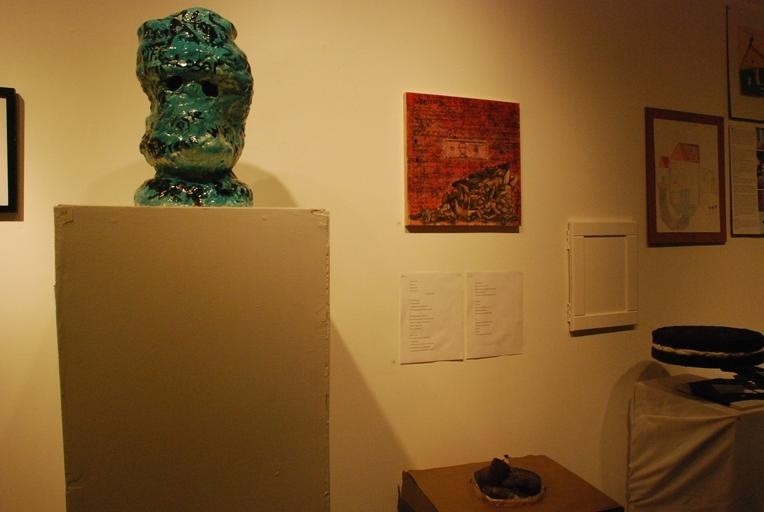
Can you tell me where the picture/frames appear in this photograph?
[0,87,18,214]
[644,0,764,245]
